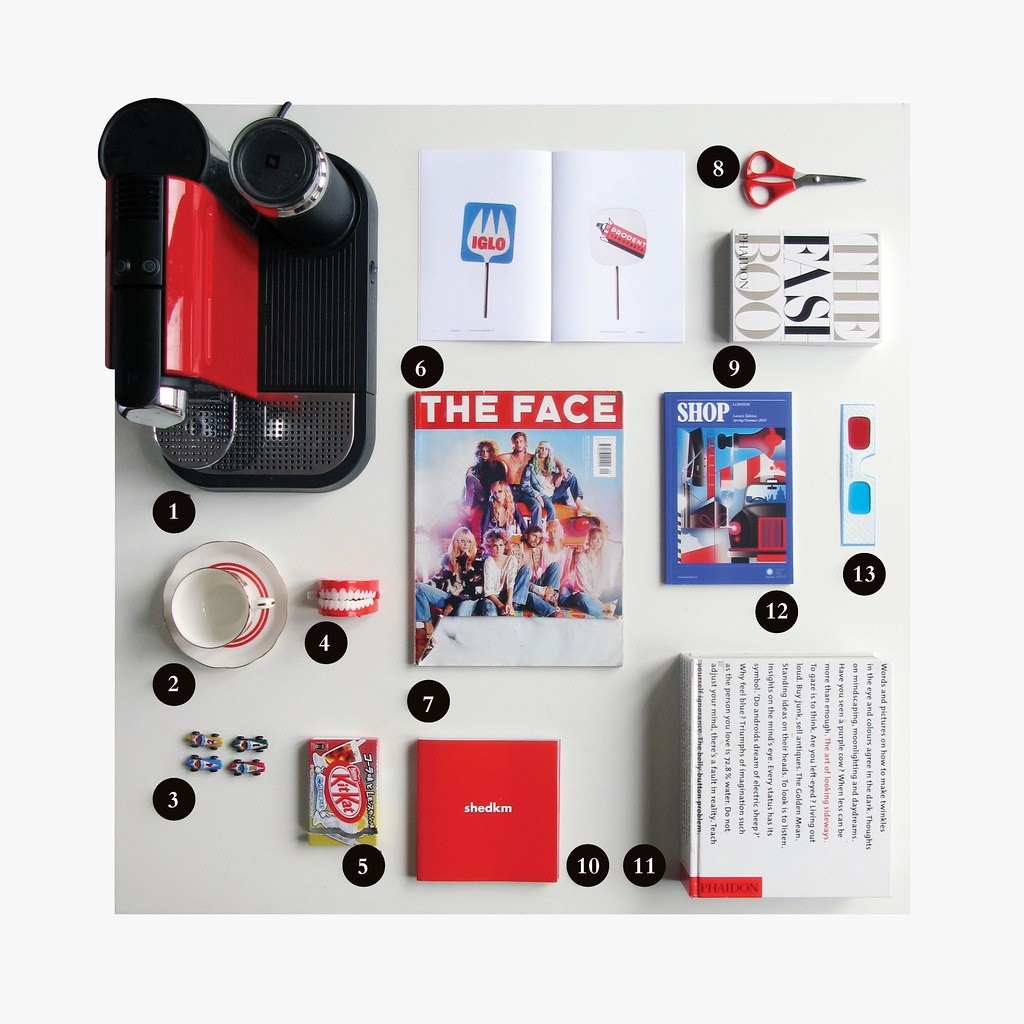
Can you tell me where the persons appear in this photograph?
[416,432,608,638]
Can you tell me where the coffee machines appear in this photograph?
[97,99,375,493]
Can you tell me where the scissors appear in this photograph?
[741,149,866,209]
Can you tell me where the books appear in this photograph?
[409,391,621,671]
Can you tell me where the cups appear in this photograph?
[172,568,274,650]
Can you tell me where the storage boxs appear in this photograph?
[676,650,892,902]
[728,227,881,343]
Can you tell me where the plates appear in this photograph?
[164,541,288,669]
